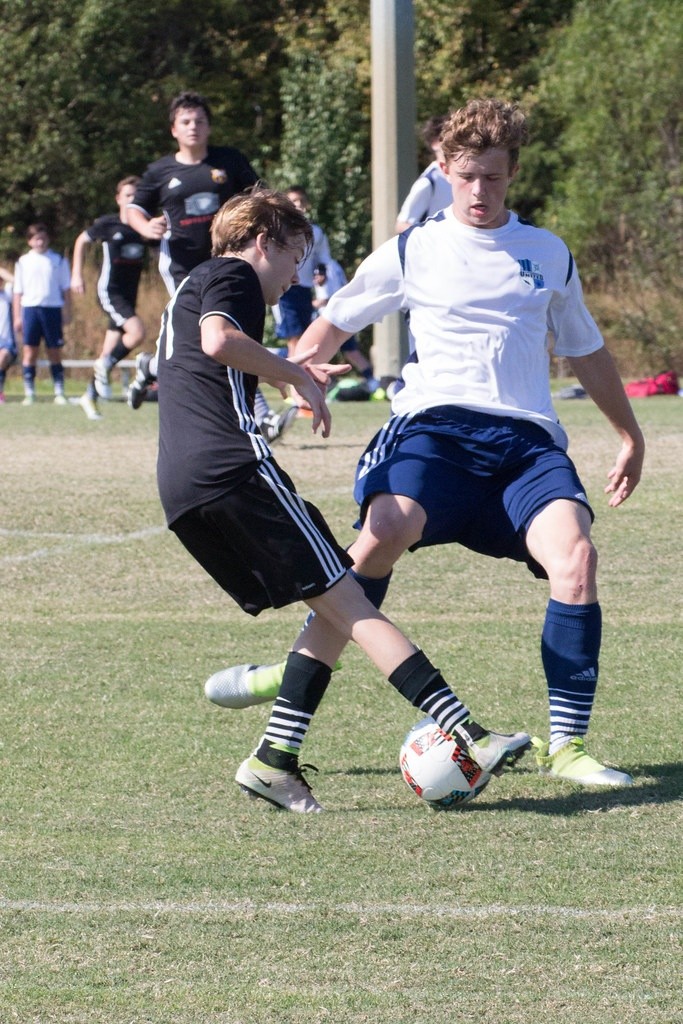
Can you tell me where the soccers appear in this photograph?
[398,716,492,808]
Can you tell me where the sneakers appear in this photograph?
[127,376,148,410]
[259,405,301,445]
[22,395,34,407]
[134,351,157,393]
[233,732,327,817]
[53,395,67,406]
[92,358,113,399]
[204,653,345,710]
[79,393,103,419]
[530,736,634,786]
[448,714,532,774]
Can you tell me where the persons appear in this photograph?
[70,176,149,418]
[12,226,72,404]
[156,190,532,813]
[394,114,453,230]
[271,184,371,379]
[126,94,299,445]
[0,265,18,404]
[206,99,645,786]
